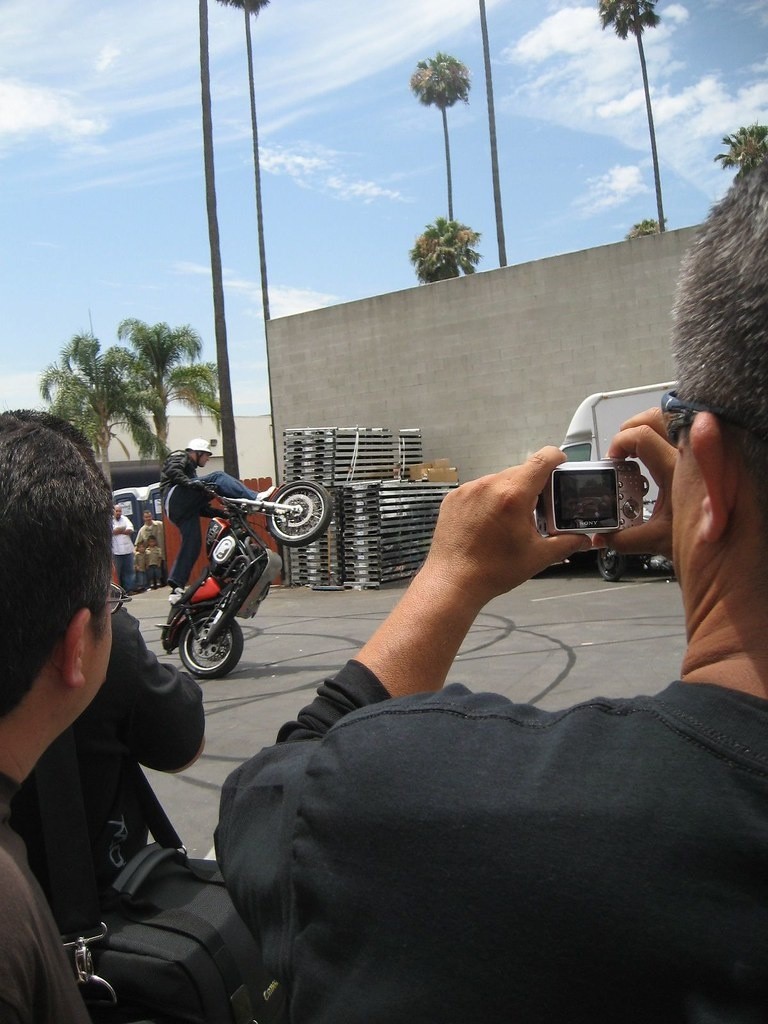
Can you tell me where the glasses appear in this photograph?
[56,581,132,637]
[661,391,768,447]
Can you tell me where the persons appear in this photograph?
[212,154,768,1024]
[562,490,612,524]
[0,404,278,1024]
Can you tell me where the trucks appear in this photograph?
[557,381,685,582]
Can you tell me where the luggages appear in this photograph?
[62,841,262,1024]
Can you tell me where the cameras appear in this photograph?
[544,458,650,535]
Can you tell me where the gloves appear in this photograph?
[181,479,203,493]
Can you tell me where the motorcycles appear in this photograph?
[154,472,333,680]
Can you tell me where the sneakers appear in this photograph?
[251,486,276,512]
[168,588,185,605]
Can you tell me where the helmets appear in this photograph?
[185,439,212,457]
